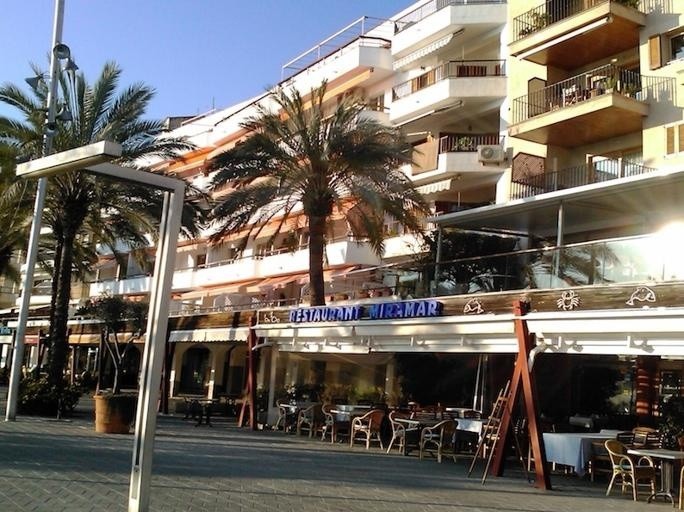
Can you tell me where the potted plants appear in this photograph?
[74,294,151,434]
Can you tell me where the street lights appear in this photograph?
[4,0,77,422]
[14,138,188,511]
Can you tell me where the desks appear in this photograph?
[627,449,684,509]
[177,394,239,427]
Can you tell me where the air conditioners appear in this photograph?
[477,144,504,162]
